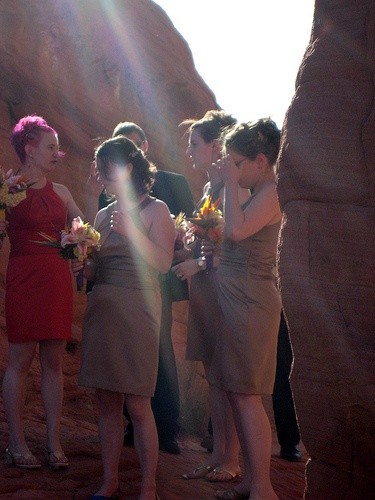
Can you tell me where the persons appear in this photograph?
[211,116,284,500]
[98,121,199,455]
[273,312,305,464]
[1,114,88,468]
[173,110,252,486]
[69,136,175,500]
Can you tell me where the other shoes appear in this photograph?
[279,449,301,462]
[124,423,133,445]
[89,486,120,500]
[164,437,180,455]
[214,487,248,499]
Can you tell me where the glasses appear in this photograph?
[232,157,246,166]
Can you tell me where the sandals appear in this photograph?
[182,465,215,479]
[205,467,241,482]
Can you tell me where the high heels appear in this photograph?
[201,435,213,454]
[44,446,70,466]
[6,448,41,468]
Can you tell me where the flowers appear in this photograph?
[170,209,198,253]
[0,164,39,209]
[184,193,227,278]
[31,215,103,293]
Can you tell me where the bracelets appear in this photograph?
[197,257,207,274]
